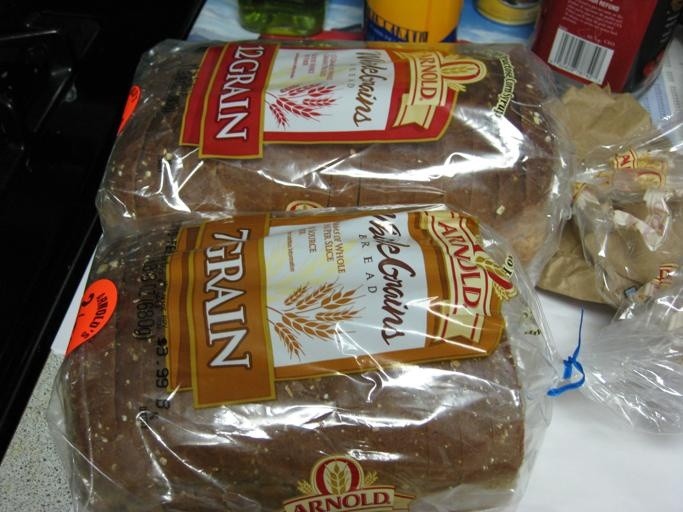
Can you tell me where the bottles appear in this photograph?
[364,0,461,43]
[238,0,325,35]
[455,0,542,43]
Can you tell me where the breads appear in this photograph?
[60,203,526,511]
[102,44,557,227]
